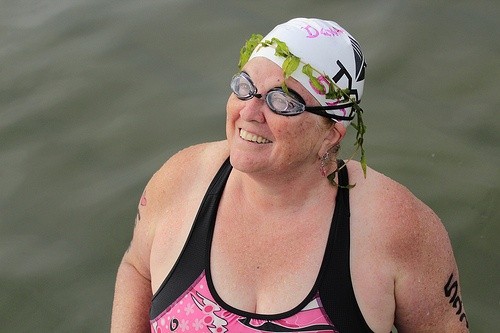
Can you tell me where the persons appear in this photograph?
[110,18,469,332]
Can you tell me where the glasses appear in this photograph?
[231,70,361,120]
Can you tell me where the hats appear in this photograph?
[248,17,366,126]
